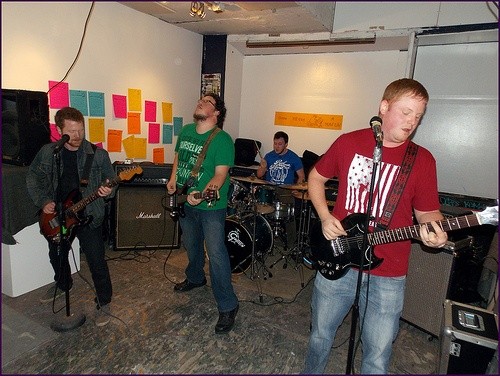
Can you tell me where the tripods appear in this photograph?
[230,180,321,288]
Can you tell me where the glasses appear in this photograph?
[197,99,217,110]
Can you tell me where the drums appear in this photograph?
[203,210,274,275]
[265,201,295,222]
[227,180,250,209]
[248,187,277,213]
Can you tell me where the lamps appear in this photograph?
[189,1,223,19]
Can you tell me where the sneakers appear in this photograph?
[214,303,239,334]
[173,277,207,293]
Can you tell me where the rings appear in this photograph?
[426,240,430,242]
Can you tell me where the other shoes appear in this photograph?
[39,285,73,302]
[95,302,113,326]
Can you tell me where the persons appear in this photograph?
[302,78,447,374]
[257,131,305,204]
[166,91,240,335]
[27,106,119,326]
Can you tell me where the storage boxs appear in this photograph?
[437,299,500,376]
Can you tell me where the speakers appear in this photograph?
[400,239,484,339]
[1,89,50,166]
[115,187,179,249]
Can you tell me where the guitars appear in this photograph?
[167,184,221,224]
[309,199,499,281]
[39,165,143,244]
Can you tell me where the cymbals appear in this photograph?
[278,182,329,190]
[291,190,311,201]
[230,175,274,185]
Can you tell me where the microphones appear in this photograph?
[370,116,384,145]
[54,135,70,152]
